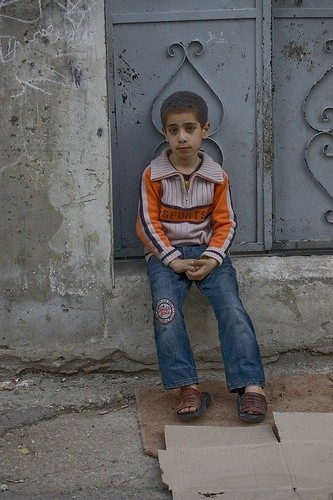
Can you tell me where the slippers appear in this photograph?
[236,390,267,423]
[176,387,212,417]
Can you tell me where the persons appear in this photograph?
[136,91,267,425]
